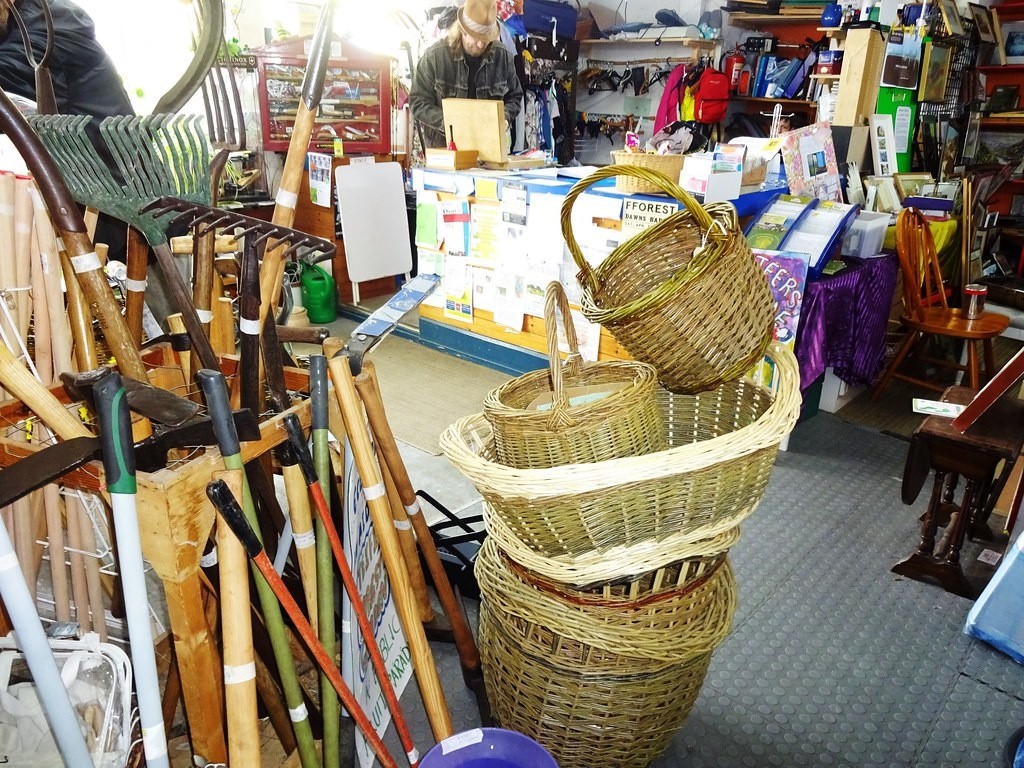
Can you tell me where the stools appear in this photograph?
[890,385,1024,601]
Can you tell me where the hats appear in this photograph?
[457,0,500,41]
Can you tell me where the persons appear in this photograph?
[0,2,191,335]
[408,0,523,149]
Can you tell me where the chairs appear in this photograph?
[869,207,1010,402]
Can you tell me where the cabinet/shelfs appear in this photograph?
[727,12,822,105]
[292,154,397,305]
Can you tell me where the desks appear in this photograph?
[413,168,686,378]
[335,161,413,307]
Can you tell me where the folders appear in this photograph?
[761,51,819,99]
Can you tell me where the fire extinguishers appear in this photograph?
[718,42,748,90]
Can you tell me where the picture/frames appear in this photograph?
[903,196,955,211]
[990,21,1024,65]
[917,42,954,102]
[936,0,966,37]
[893,171,934,200]
[967,2,997,45]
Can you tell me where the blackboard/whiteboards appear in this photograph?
[334,162,413,283]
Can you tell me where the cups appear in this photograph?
[962,284,987,320]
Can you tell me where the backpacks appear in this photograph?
[694,67,729,124]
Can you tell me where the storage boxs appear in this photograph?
[442,98,544,171]
[816,50,844,75]
[841,210,892,257]
[425,147,479,171]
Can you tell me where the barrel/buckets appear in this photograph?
[417,727,559,768]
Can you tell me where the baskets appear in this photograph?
[483,282,666,469]
[440,344,804,768]
[561,163,775,396]
[612,150,685,194]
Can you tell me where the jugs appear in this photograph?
[299,259,337,323]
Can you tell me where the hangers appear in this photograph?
[530,57,713,96]
[587,112,644,146]
